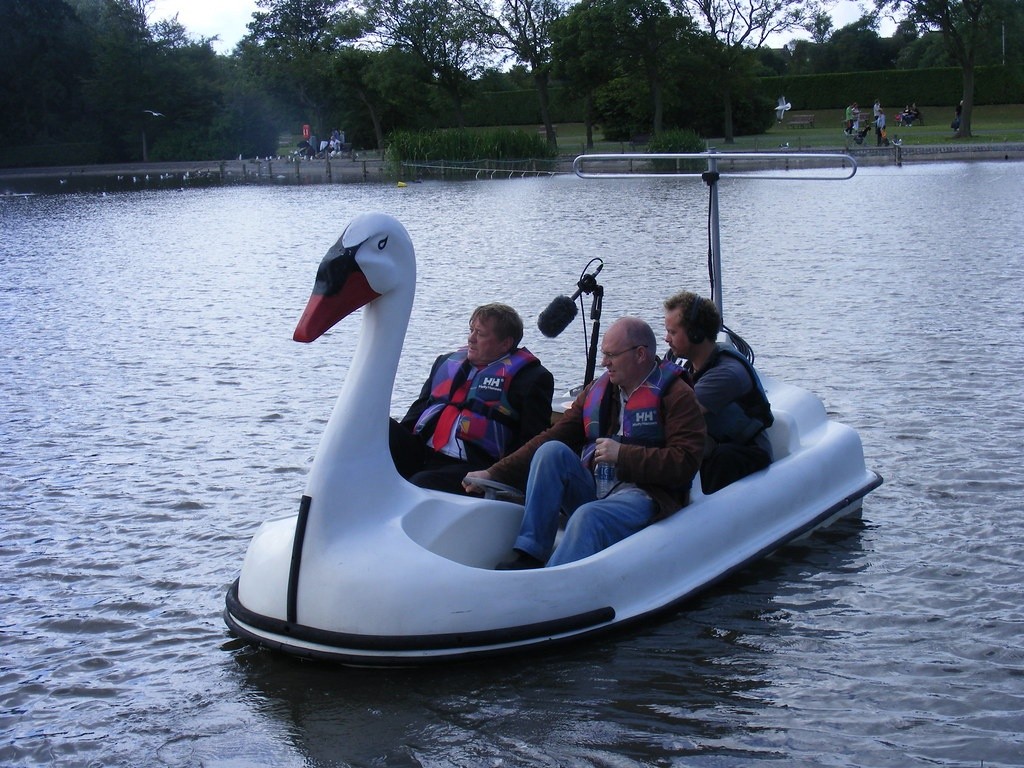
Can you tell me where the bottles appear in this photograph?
[594,455,618,501]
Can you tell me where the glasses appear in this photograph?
[600,344,649,358]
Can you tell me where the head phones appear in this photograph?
[687,291,707,346]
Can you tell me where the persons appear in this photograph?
[899,104,912,127]
[871,107,890,147]
[462,315,708,570]
[905,102,918,127]
[851,104,860,134]
[879,125,890,146]
[292,130,349,162]
[390,303,555,496]
[873,98,881,133]
[662,291,773,501]
[954,100,964,132]
[844,102,854,136]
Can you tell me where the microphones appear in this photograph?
[536,261,604,339]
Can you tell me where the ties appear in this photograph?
[432,363,488,452]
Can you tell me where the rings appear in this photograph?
[597,450,600,456]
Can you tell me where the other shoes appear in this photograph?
[497,554,542,570]
